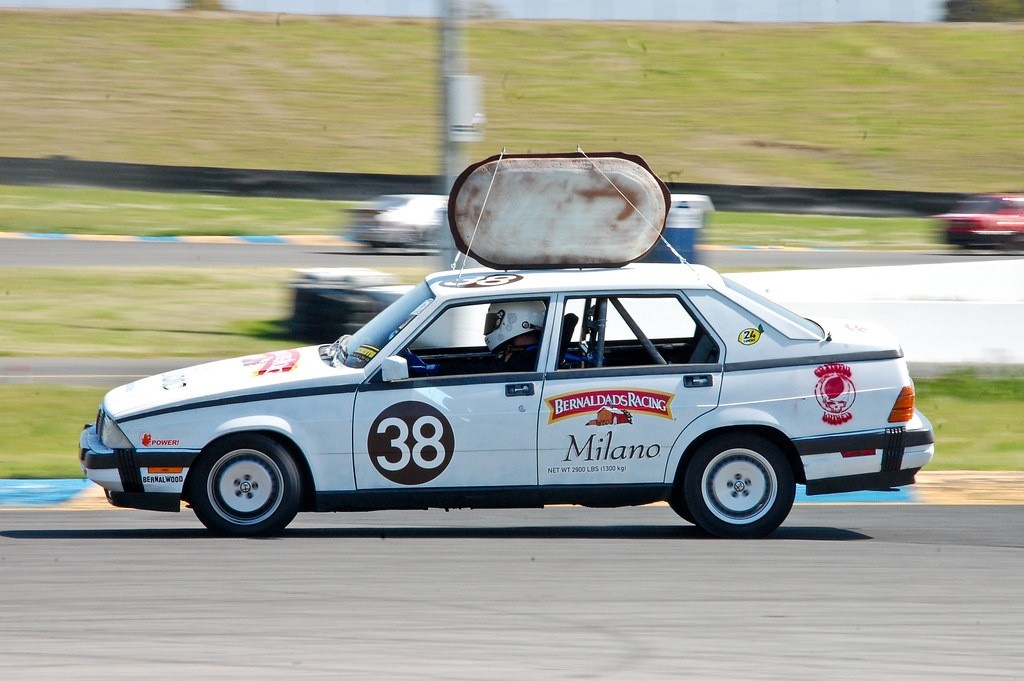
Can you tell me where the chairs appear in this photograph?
[559,312,578,367]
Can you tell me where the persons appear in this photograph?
[402,300,546,377]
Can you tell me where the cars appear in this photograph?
[350,193,447,249]
[945,194,1024,252]
[77,264,936,541]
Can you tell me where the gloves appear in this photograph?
[401,351,426,374]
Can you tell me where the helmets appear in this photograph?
[484,300,547,354]
